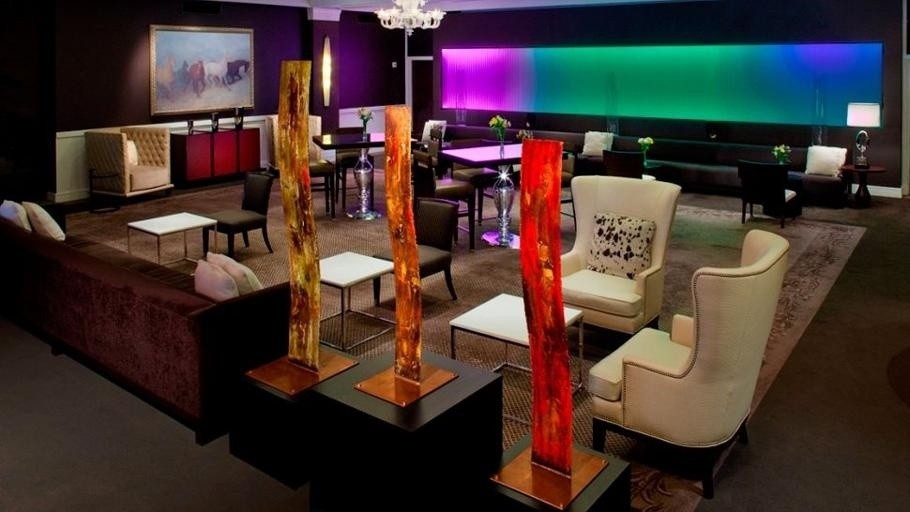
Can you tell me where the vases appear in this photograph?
[499,139,505,153]
[362,123,367,134]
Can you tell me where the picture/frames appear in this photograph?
[147,23,256,118]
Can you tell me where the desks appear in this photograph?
[449,292,588,430]
[841,165,889,205]
[319,250,396,353]
[313,133,419,223]
[309,342,507,508]
[126,211,218,277]
[472,429,634,510]
[227,343,366,493]
[439,140,571,248]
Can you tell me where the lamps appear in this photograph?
[373,0,448,39]
[321,34,333,108]
[846,102,883,169]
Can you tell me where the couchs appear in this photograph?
[427,104,855,214]
[558,174,686,341]
[265,114,322,168]
[586,229,793,501]
[0,198,300,446]
[84,124,175,200]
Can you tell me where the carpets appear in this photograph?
[57,161,870,510]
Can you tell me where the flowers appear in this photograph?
[637,136,655,159]
[768,142,793,165]
[356,106,374,142]
[488,113,513,143]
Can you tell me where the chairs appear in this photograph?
[603,150,665,181]
[419,118,450,146]
[451,138,506,226]
[736,159,797,229]
[202,173,275,256]
[411,129,444,179]
[554,156,608,231]
[330,125,379,211]
[411,148,477,252]
[371,197,459,308]
[310,158,335,221]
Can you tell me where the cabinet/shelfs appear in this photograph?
[231,128,262,177]
[202,127,239,181]
[171,129,212,182]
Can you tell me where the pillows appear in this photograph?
[126,140,140,166]
[587,129,615,152]
[205,250,265,297]
[0,200,34,233]
[582,131,610,157]
[585,208,658,280]
[21,200,67,243]
[812,145,849,176]
[804,145,841,177]
[194,259,241,303]
